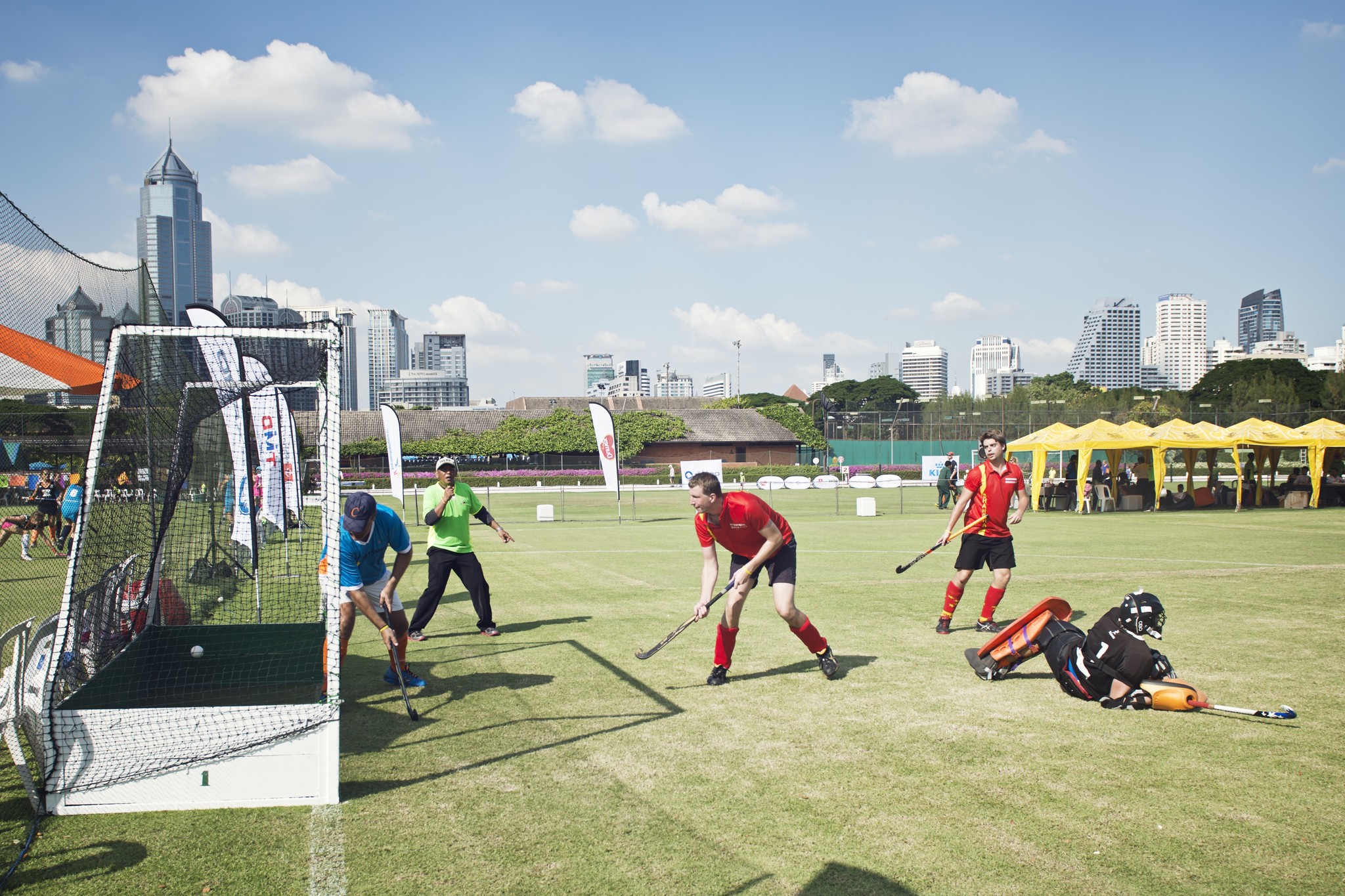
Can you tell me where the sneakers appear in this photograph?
[707,663,727,686]
[408,630,426,641]
[57,536,65,551]
[383,662,427,687]
[975,617,1004,633]
[20,553,36,561]
[936,616,951,634]
[816,645,838,676]
[481,627,500,636]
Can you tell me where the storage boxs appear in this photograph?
[1227,491,1237,505]
[1284,490,1311,510]
[1122,485,1139,495]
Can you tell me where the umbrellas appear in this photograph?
[1,322,142,397]
[28,461,67,469]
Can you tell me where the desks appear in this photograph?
[1026,485,1067,512]
[1298,482,1345,508]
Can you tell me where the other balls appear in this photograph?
[125,549,131,555]
[217,596,225,603]
[190,645,205,658]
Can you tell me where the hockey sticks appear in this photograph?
[1185,695,1297,720]
[634,578,740,660]
[895,514,990,574]
[14,493,58,501]
[55,551,108,559]
[379,593,421,722]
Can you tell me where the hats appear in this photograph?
[343,492,376,532]
[436,457,456,471]
[946,451,954,456]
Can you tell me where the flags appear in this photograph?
[589,401,619,492]
[184,302,304,555]
[317,378,325,431]
[379,403,404,507]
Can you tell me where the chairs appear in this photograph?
[1094,484,1117,512]
[1232,479,1244,491]
[92,488,159,505]
[1,552,143,785]
[0,615,47,816]
[1214,481,1224,506]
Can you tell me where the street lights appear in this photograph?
[891,398,930,467]
[983,393,1008,441]
[1257,399,1280,423]
[1199,403,1218,426]
[1132,395,1161,425]
[1029,399,1066,426]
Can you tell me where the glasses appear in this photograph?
[947,454,951,456]
[1010,459,1013,461]
[42,473,50,476]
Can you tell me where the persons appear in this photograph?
[0,469,85,561]
[936,451,1345,510]
[688,471,838,686]
[403,457,514,641]
[965,585,1208,712]
[936,427,1030,635]
[739,472,746,491]
[668,465,676,486]
[316,490,428,712]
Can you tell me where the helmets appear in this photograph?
[1120,585,1167,640]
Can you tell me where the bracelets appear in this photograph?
[742,566,752,575]
[379,625,389,631]
[496,527,503,531]
[441,496,448,504]
[944,530,952,534]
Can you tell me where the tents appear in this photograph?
[1001,417,1345,515]
